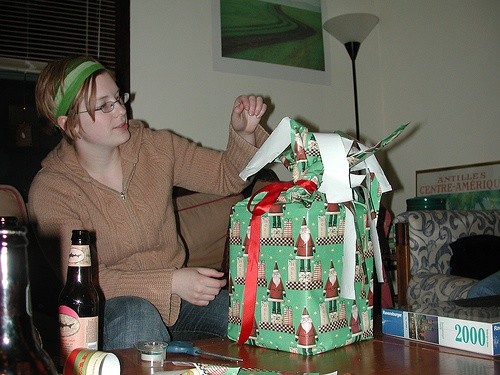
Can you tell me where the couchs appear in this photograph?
[0,178,271,353]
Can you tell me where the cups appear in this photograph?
[136,340,168,368]
[63,348,121,375]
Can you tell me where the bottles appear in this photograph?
[58,230,100,372]
[0,215,58,375]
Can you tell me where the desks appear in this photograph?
[100,315,500,375]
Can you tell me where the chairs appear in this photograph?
[393,210,500,309]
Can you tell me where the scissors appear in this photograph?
[166,340,243,362]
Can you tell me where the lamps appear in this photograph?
[324,13,380,150]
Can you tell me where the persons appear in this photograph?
[28,52,270,351]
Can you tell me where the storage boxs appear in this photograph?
[382,302,500,357]
[227,115,410,356]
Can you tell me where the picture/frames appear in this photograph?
[210,0,332,86]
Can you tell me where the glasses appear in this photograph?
[77,92,130,114]
